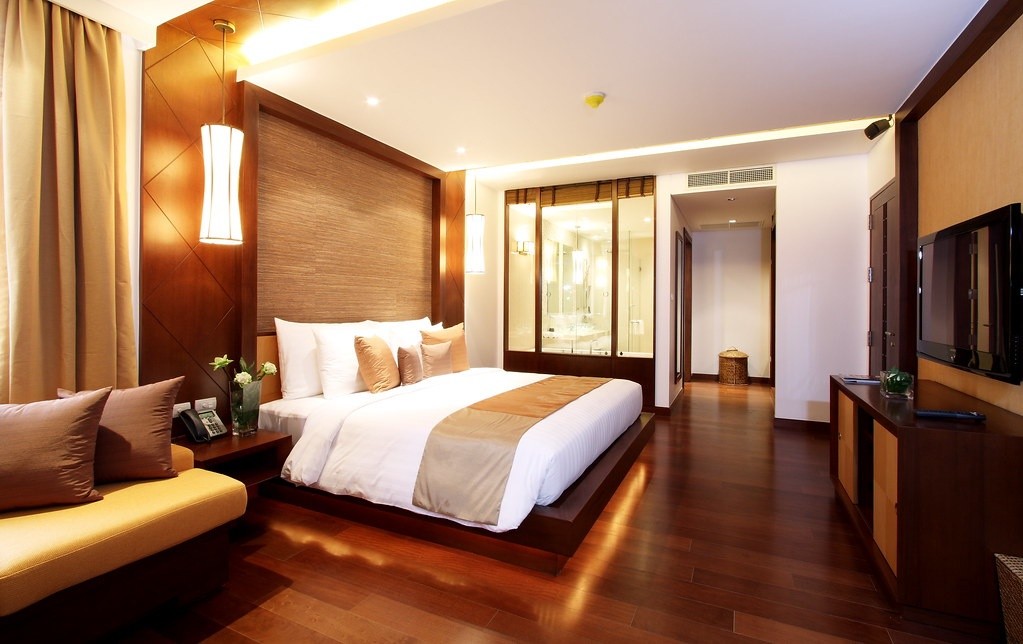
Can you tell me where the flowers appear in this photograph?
[208,354,278,424]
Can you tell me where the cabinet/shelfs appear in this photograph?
[829,376,1023,623]
[868,177,897,375]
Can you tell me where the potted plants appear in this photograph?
[879,365,915,400]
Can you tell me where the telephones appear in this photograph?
[179,408,227,443]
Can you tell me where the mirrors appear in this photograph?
[546,238,588,315]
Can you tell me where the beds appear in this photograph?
[258,331,655,557]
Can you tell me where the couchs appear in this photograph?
[0,442,248,644]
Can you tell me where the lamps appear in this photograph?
[464,174,486,273]
[571,225,584,284]
[517,240,535,255]
[201,19,244,248]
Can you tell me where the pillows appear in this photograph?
[56,376,183,479]
[274,316,471,401]
[0,385,113,510]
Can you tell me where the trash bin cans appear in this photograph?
[718,345,748,386]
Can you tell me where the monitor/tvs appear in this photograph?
[918,203,1023,385]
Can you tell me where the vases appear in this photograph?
[228,380,262,437]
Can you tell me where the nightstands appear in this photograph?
[174,423,293,488]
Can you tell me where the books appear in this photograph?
[840,373,881,384]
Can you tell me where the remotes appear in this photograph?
[913,410,985,422]
